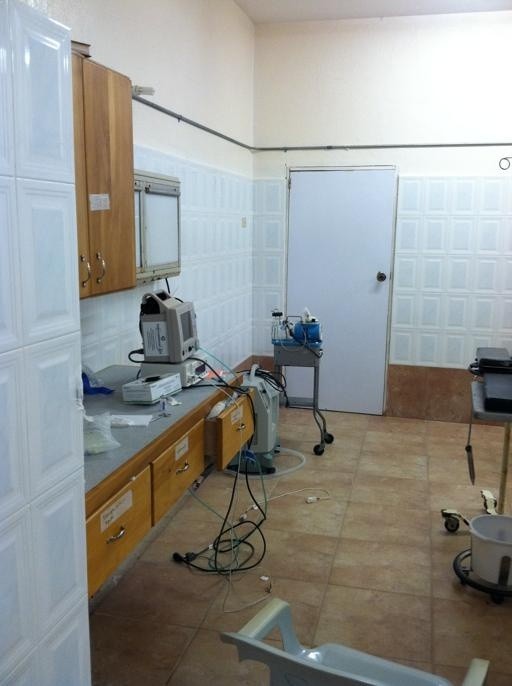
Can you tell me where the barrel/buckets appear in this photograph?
[468,515,512,586]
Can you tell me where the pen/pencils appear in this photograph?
[151,416,160,422]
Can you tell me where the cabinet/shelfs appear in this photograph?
[0,1,92,685]
[83,365,255,600]
[71,49,137,300]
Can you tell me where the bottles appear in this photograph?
[271,312,284,340]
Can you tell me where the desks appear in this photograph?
[441,381,512,531]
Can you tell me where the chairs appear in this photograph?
[220,595,490,686]
